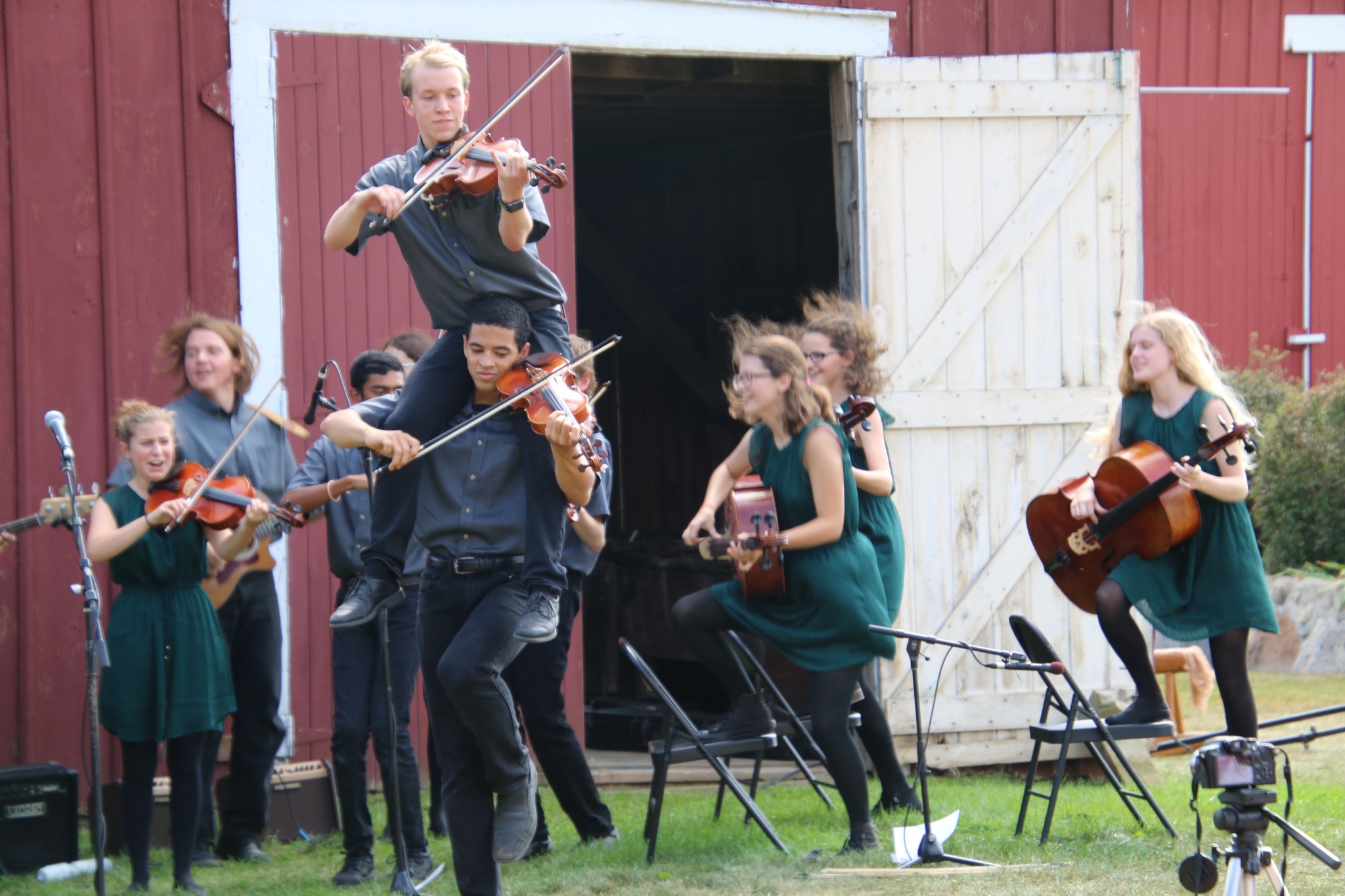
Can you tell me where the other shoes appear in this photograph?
[332,849,375,887]
[239,841,270,863]
[193,852,220,867]
[1104,696,1171,725]
[428,805,448,839]
[117,882,151,896]
[870,783,922,816]
[577,826,620,851]
[172,870,207,896]
[795,680,864,718]
[518,835,554,861]
[385,846,434,889]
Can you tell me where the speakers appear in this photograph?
[87,772,215,858]
[0,760,82,878]
[219,758,343,846]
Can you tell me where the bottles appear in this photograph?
[37,857,112,881]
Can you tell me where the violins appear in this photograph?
[412,131,567,202]
[142,461,310,530]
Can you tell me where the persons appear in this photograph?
[84,398,268,892]
[106,303,303,869]
[281,295,615,896]
[672,287,921,856]
[1070,305,1281,739]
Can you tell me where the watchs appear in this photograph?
[500,197,522,211]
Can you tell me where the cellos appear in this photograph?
[1025,413,1261,615]
[834,393,879,442]
[696,470,788,603]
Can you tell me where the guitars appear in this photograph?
[495,355,604,473]
[0,484,101,542]
[196,503,329,611]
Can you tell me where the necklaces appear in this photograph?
[323,38,581,642]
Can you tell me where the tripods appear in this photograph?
[867,623,1027,868]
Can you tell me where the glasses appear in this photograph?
[732,371,773,392]
[802,350,841,364]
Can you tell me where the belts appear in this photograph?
[423,553,527,575]
[546,304,562,315]
[397,576,421,587]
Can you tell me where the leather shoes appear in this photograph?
[492,757,538,863]
[513,591,560,643]
[328,575,405,629]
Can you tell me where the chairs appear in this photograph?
[1008,613,1181,845]
[621,638,791,865]
[714,626,861,827]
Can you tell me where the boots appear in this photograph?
[830,821,883,859]
[698,688,777,740]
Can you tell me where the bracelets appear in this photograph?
[573,505,582,513]
[327,478,341,502]
[700,504,718,513]
[144,513,155,528]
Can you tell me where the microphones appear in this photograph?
[44,407,77,461]
[303,361,327,424]
[984,660,1065,675]
